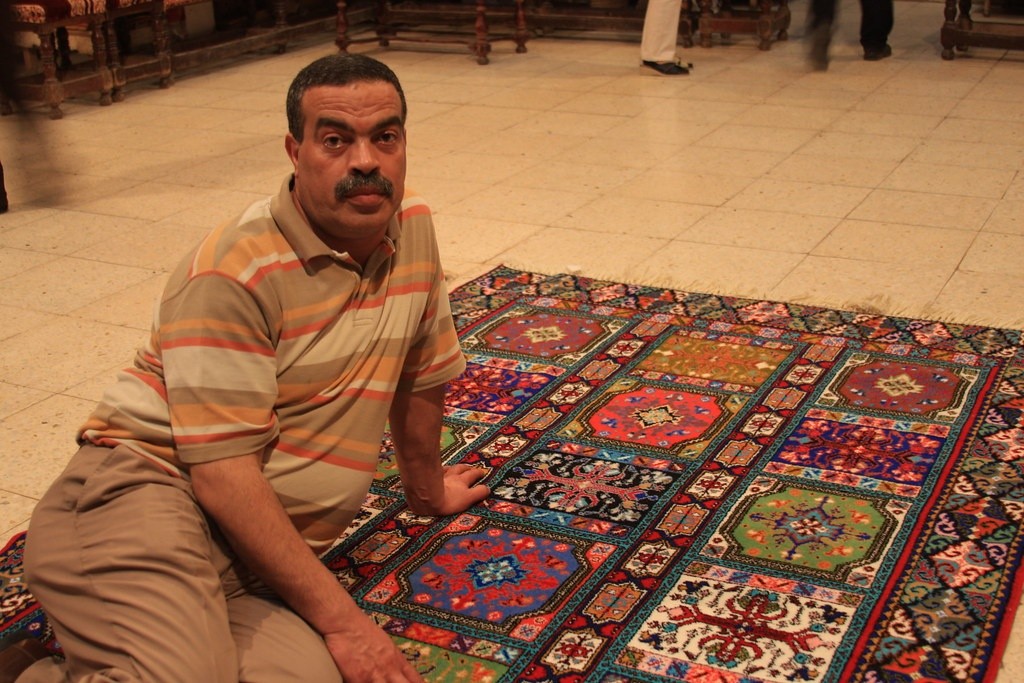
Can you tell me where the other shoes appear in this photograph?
[861,43,893,62]
[803,49,831,73]
[641,53,693,76]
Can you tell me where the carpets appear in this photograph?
[0,266,1024,683]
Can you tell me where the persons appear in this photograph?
[801,0,894,72]
[641,0,694,75]
[0,53,490,683]
